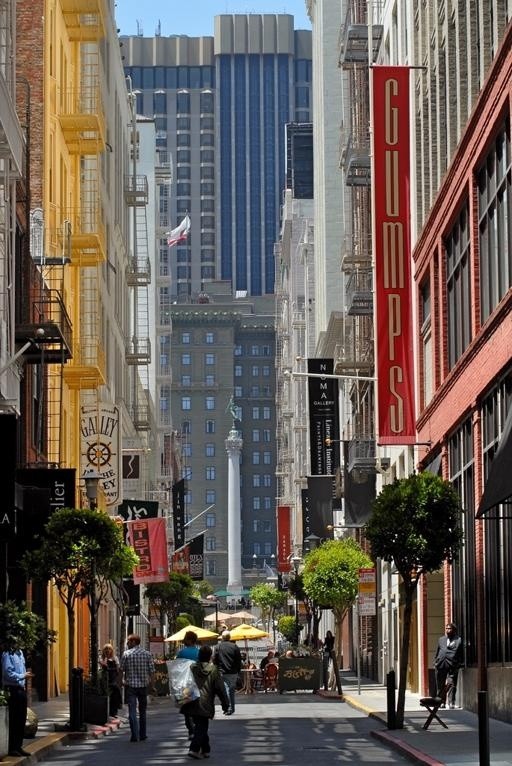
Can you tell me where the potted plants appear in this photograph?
[0,599,58,758]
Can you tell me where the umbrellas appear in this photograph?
[231,611,257,619]
[239,589,252,595]
[217,623,270,668]
[204,612,231,620]
[212,589,237,597]
[163,624,220,642]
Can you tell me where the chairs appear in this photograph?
[250,663,277,694]
[419,683,452,729]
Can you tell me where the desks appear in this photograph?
[237,669,258,694]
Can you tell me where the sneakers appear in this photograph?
[188,752,209,759]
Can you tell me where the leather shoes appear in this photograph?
[9,748,31,756]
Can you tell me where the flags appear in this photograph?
[128,518,169,586]
[165,216,191,248]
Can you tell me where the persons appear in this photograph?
[188,645,230,759]
[177,631,199,740]
[216,596,251,610]
[213,631,241,712]
[1,648,31,757]
[433,623,463,709]
[99,644,120,718]
[240,630,335,691]
[118,634,155,742]
